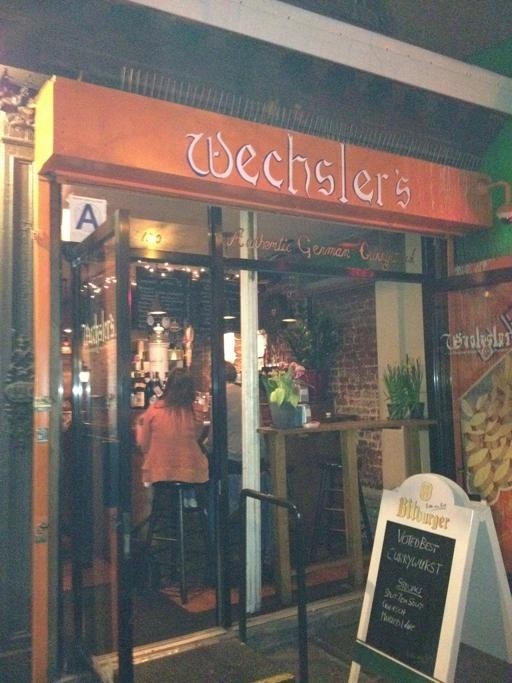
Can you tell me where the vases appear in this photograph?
[267,397,305,430]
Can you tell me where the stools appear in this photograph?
[138,474,224,601]
[314,452,375,558]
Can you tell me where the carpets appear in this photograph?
[62,548,123,593]
[154,555,414,612]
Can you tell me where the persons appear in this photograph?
[205,361,269,475]
[135,366,209,539]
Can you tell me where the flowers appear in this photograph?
[266,365,308,405]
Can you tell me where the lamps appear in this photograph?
[79,362,91,392]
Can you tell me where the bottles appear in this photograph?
[132,371,168,410]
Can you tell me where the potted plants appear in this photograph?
[383,352,426,421]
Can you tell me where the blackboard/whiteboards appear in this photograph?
[133,264,240,332]
[365,521,456,679]
